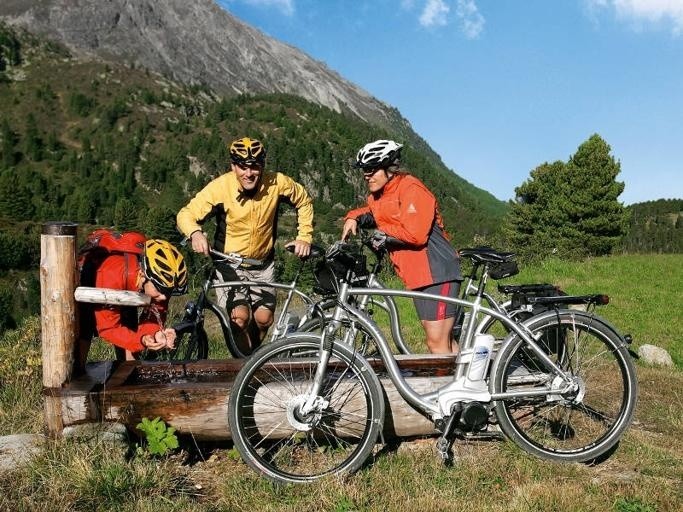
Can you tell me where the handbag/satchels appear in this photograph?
[314,240,368,292]
[513,283,571,361]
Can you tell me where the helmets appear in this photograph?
[229,136,267,167]
[355,139,404,171]
[142,238,188,297]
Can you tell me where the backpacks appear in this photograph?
[76,227,148,291]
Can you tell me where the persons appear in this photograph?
[339,138,465,357]
[176,138,315,358]
[73,227,188,365]
